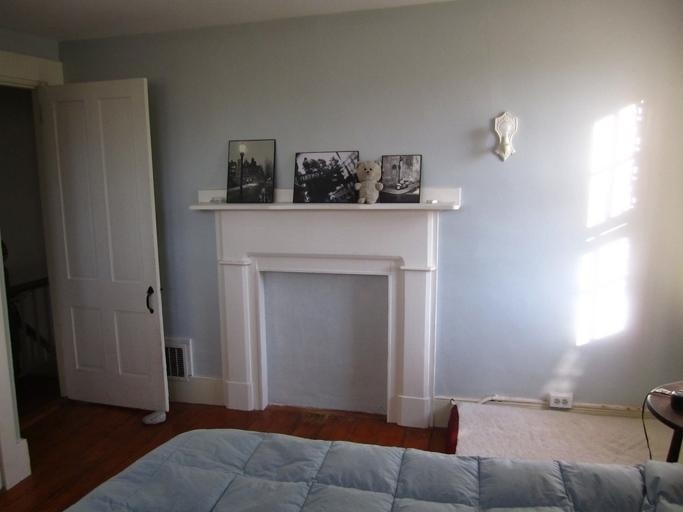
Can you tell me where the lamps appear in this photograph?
[494,111,518,161]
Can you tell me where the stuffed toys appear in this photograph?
[355,160,384,203]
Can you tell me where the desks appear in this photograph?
[646,380,683,463]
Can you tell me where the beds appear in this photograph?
[60,428,683,512]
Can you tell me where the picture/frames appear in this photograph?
[292,151,359,204]
[227,139,276,204]
[379,154,422,204]
